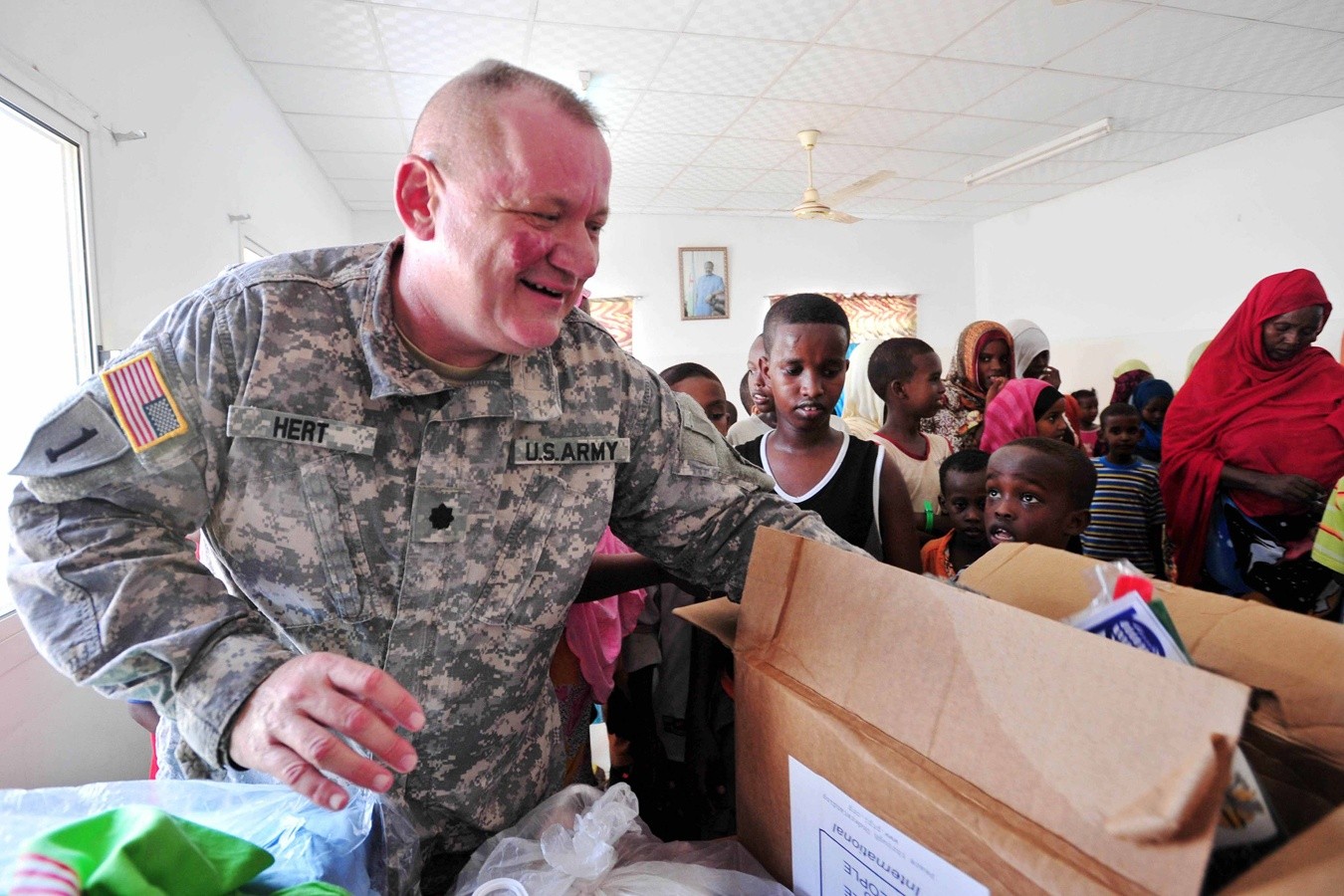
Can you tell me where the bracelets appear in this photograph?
[710,293,714,298]
[923,500,934,534]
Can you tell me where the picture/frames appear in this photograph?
[677,247,729,321]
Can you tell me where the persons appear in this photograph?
[5,60,998,896]
[691,261,725,316]
[551,294,1173,846]
[1159,269,1344,619]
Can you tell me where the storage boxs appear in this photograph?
[670,523,1344,896]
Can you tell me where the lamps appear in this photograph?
[963,117,1115,187]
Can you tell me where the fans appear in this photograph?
[695,131,896,223]
